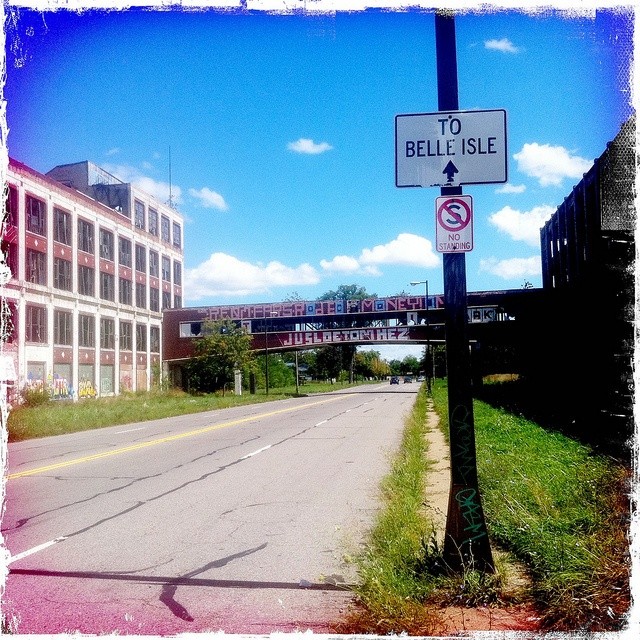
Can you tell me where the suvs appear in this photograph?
[404,377,412,383]
[390,377,399,385]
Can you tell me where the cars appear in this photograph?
[418,377,425,382]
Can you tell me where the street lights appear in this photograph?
[411,281,432,392]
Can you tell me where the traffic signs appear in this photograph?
[395,110,507,187]
